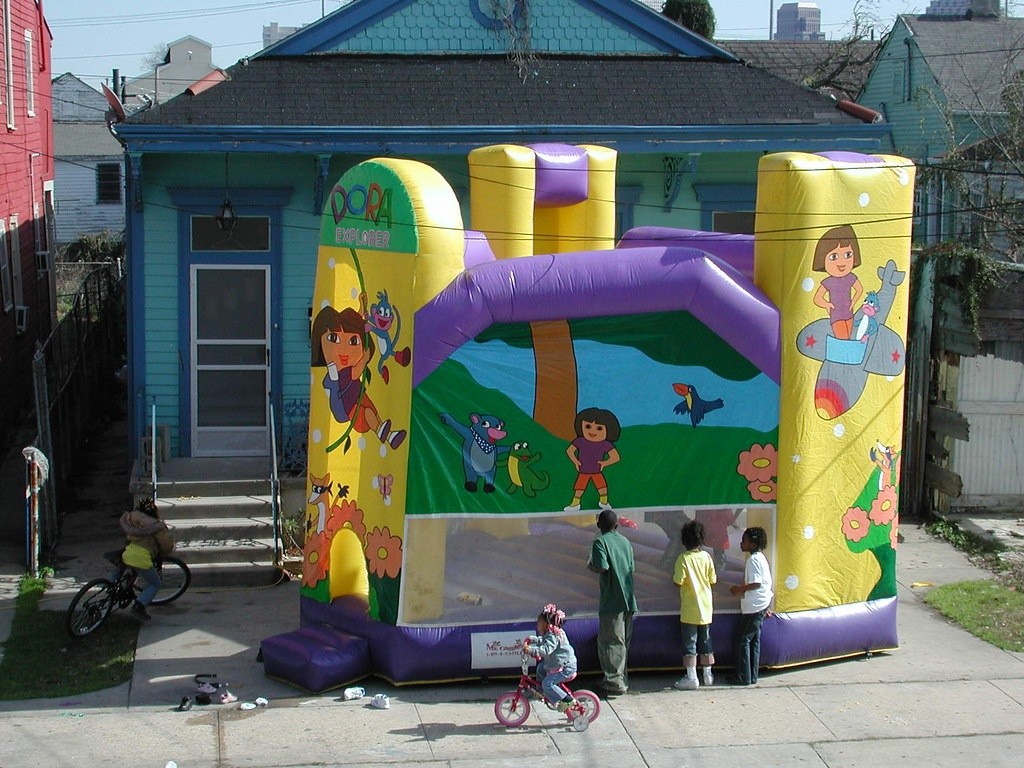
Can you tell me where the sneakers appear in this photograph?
[371,694,390,709]
[724,675,756,684]
[589,683,624,699]
[344,687,366,700]
[196,683,217,694]
[220,690,238,703]
[131,604,151,621]
[179,696,192,710]
[704,675,714,685]
[673,675,699,689]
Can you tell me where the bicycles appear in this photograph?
[495,640,603,733]
[62,545,191,639]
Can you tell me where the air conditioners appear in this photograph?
[15,305,30,329]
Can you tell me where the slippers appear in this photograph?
[558,698,576,712]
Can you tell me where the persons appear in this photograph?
[672,521,718,689]
[102,498,172,621]
[587,511,635,694]
[522,604,577,712]
[724,527,774,685]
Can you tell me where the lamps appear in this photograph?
[214,153,239,233]
